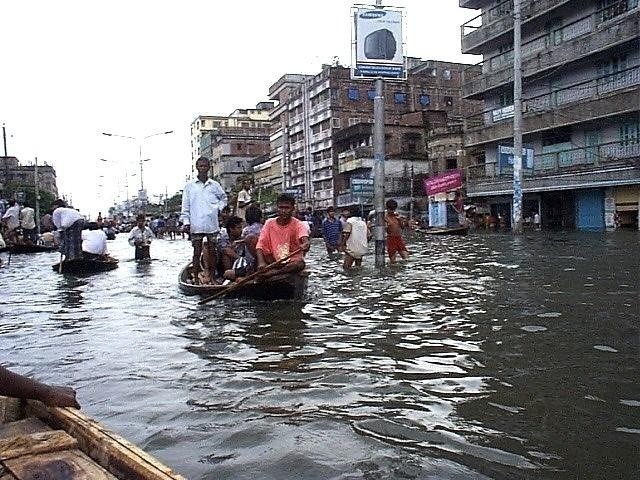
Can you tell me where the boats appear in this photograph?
[176,254,308,303]
[8,242,57,256]
[412,224,469,237]
[54,253,120,274]
[1,380,188,480]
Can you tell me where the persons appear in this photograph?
[384,200,409,264]
[199,177,310,284]
[453,191,465,227]
[296,205,325,238]
[146,213,185,241]
[394,211,429,230]
[181,158,228,284]
[523,209,541,231]
[81,222,106,258]
[1,199,61,248]
[321,204,373,270]
[614,210,624,231]
[98,212,136,240]
[128,214,154,260]
[49,199,85,260]
[466,211,510,232]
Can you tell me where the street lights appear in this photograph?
[99,157,151,218]
[102,128,174,222]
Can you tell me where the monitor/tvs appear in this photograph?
[364,30,396,60]
